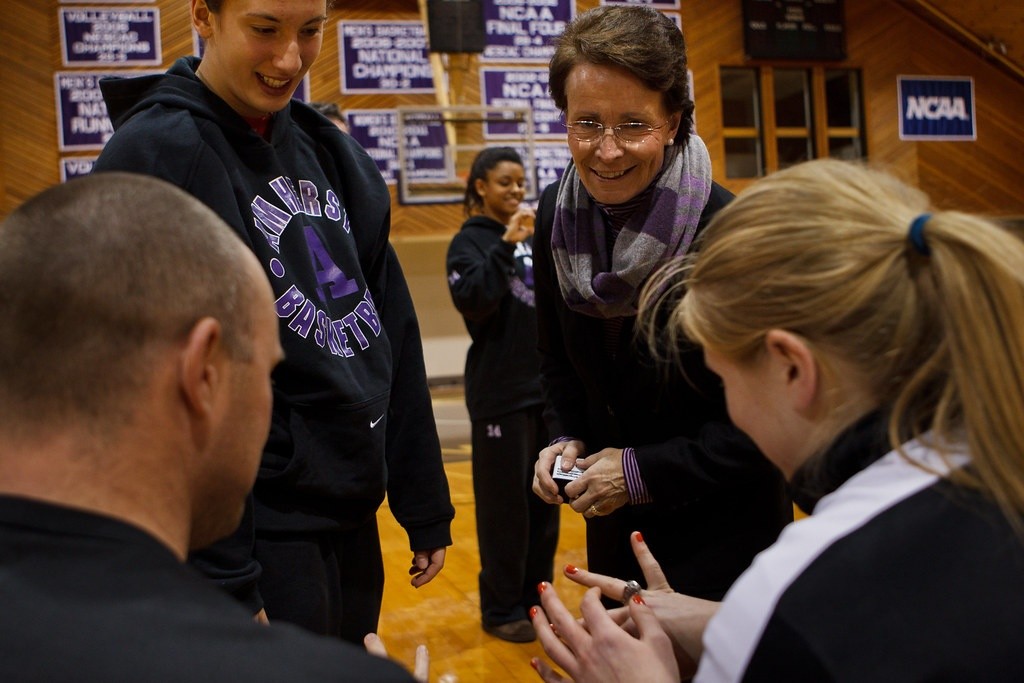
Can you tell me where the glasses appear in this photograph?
[557,111,678,141]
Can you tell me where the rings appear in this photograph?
[591,504,602,516]
[620,580,640,604]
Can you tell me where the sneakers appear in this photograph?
[491,617,536,644]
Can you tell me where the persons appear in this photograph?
[90,2,456,653]
[529,153,1024,682]
[444,147,563,644]
[0,168,464,683]
[523,3,795,607]
